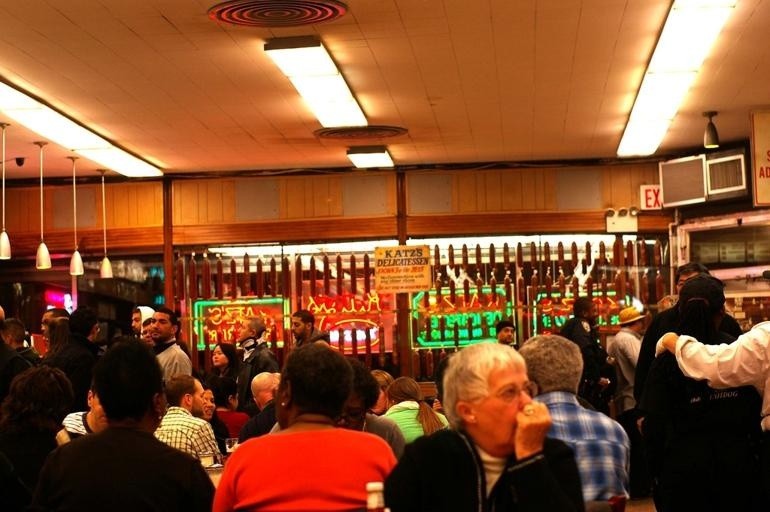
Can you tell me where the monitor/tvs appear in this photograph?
[659,154,706,208]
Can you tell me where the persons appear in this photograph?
[1,305,451,511]
[495,322,516,344]
[515,335,631,504]
[383,338,585,511]
[561,263,770,512]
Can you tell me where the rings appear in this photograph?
[525,407,535,417]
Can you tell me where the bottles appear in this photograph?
[197,450,214,469]
[365,482,387,512]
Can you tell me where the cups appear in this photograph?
[225,437,239,452]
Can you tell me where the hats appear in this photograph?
[679,273,726,310]
[615,306,645,325]
[496,321,515,332]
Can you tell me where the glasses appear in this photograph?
[337,413,365,428]
[496,381,538,402]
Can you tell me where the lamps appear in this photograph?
[263,36,368,128]
[346,146,394,169]
[0,123,11,259]
[33,142,52,269]
[615,0,736,157]
[96,169,113,279]
[0,82,163,178]
[702,110,720,149]
[67,157,84,275]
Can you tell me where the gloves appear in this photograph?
[654,332,677,357]
